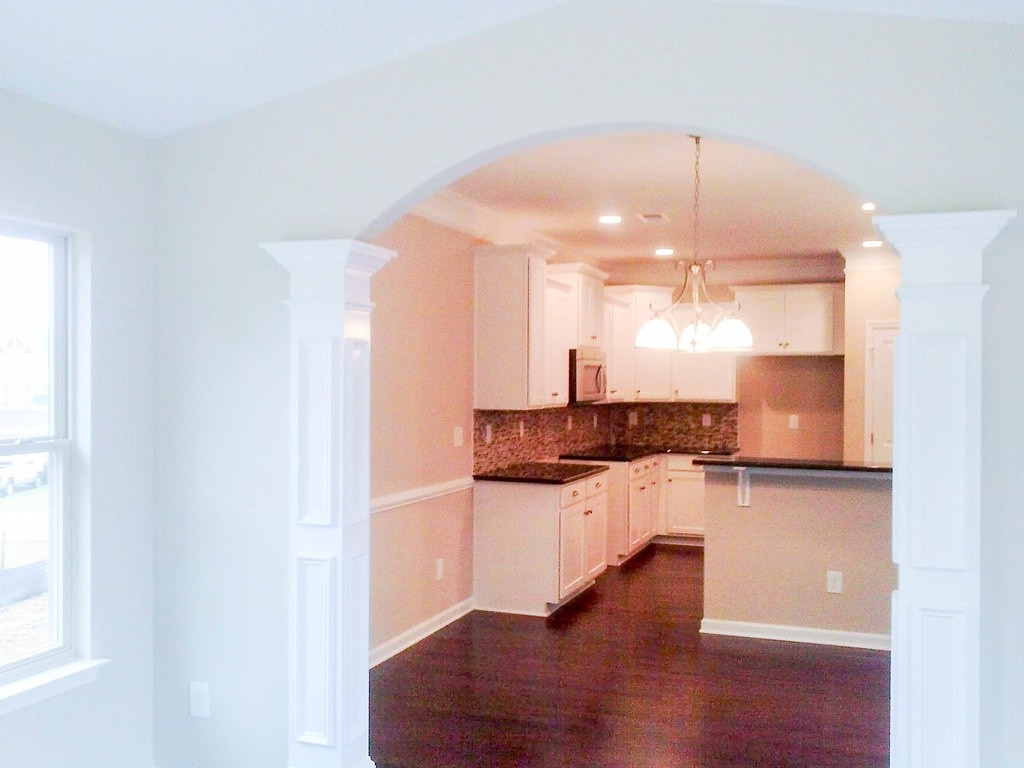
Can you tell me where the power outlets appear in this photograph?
[436,558,444,581]
[593,415,597,428]
[702,414,712,427]
[568,415,572,431]
[486,425,492,443]
[453,426,463,447]
[520,420,524,437]
[826,571,842,594]
[629,412,638,425]
[789,414,799,429]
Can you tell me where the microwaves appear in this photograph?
[576,358,606,403]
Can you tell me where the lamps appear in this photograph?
[635,135,753,354]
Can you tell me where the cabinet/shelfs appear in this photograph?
[734,286,844,355]
[545,294,569,408]
[673,302,737,404]
[651,453,705,546]
[474,471,607,617]
[474,248,545,410]
[549,270,605,350]
[559,456,659,566]
[578,303,630,406]
[606,292,672,402]
[864,322,900,468]
